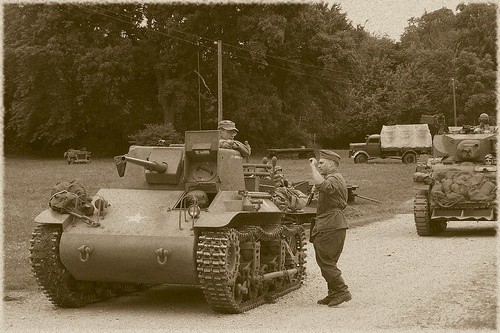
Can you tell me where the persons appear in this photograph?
[217,119,251,158]
[308,150,352,307]
[470,112,491,133]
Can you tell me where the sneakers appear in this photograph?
[328,291,352,307]
[317,295,335,305]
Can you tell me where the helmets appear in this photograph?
[478,113,489,119]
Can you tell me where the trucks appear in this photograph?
[349,124,432,165]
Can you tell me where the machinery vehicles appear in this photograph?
[413,113,497,236]
[28,129,307,313]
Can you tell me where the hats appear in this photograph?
[319,150,341,163]
[218,120,239,131]
